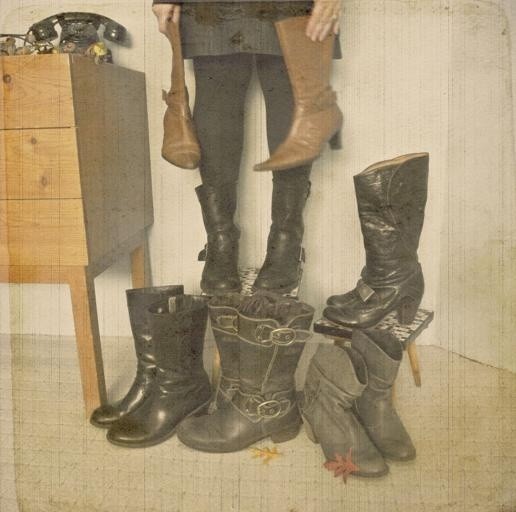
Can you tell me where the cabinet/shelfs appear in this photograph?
[0,53,155,436]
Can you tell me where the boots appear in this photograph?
[106,297,213,449]
[351,328,417,466]
[250,174,311,296]
[156,19,207,171]
[193,184,244,294]
[319,153,431,328]
[297,341,390,478]
[89,284,185,428]
[173,295,317,456]
[199,296,239,414]
[253,13,346,173]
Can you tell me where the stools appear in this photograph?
[201,266,306,397]
[314,300,433,403]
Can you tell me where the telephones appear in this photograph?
[31,12,127,65]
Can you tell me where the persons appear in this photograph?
[152,0,346,294]
